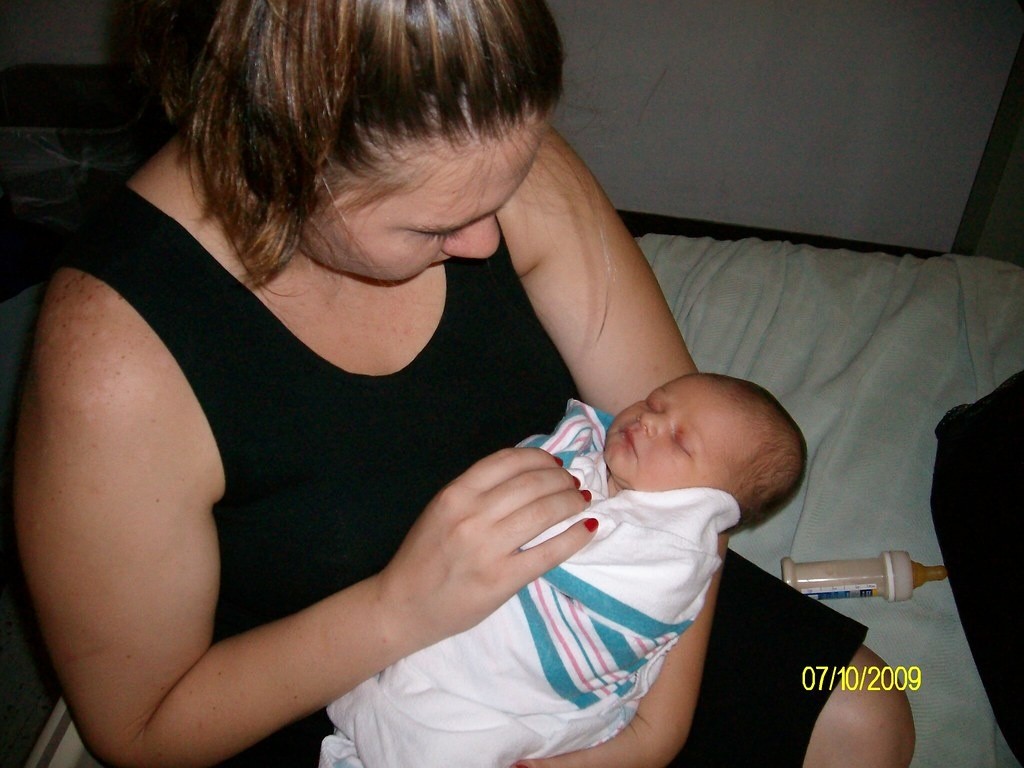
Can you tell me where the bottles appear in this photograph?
[781,550,948,603]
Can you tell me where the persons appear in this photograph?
[318,374,808,767]
[17,0,913,768]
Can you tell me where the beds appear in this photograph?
[23,212,1024,768]
